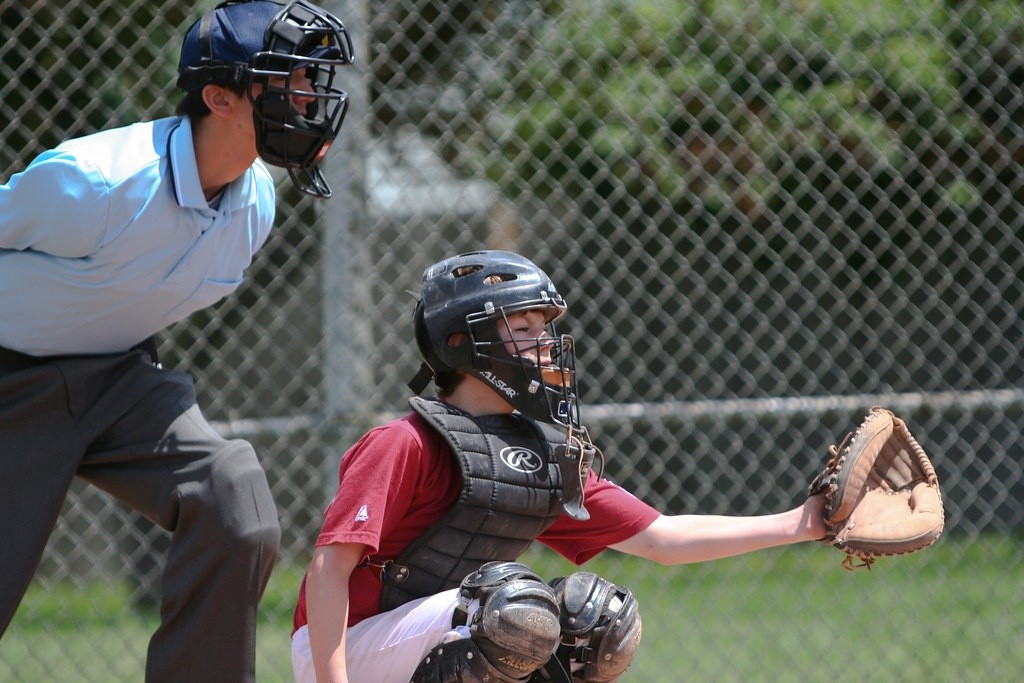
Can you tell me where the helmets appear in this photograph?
[413,251,583,433]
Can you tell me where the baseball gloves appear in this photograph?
[808,407,944,556]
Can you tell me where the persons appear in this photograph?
[290,249,826,683]
[0,0,354,683]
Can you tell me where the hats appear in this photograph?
[177,1,340,89]
